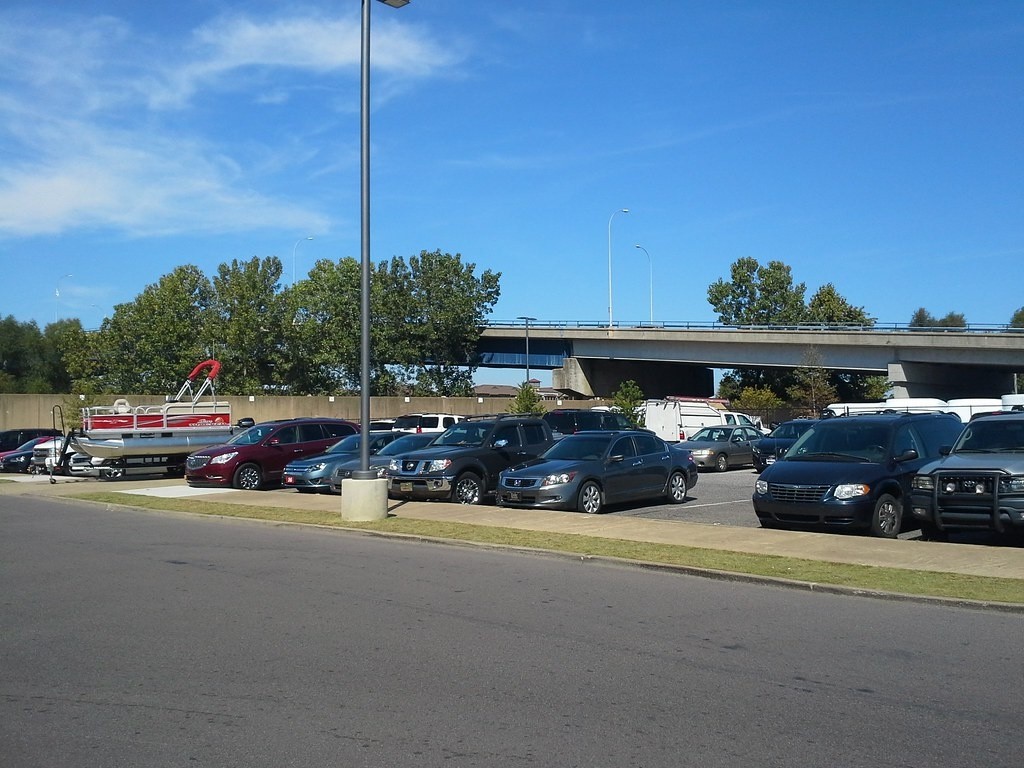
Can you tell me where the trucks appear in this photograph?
[645,399,755,444]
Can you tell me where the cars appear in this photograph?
[911,410,1024,540]
[752,418,820,472]
[281,431,413,496]
[370,419,394,432]
[393,414,465,435]
[751,408,964,540]
[671,425,766,472]
[1,429,186,481]
[498,430,699,515]
[330,433,441,499]
[186,417,360,491]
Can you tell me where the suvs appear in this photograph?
[384,413,555,505]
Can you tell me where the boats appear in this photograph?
[69,360,255,462]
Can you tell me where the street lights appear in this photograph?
[608,209,629,329]
[516,316,537,385]
[351,0,410,479]
[635,245,653,326]
